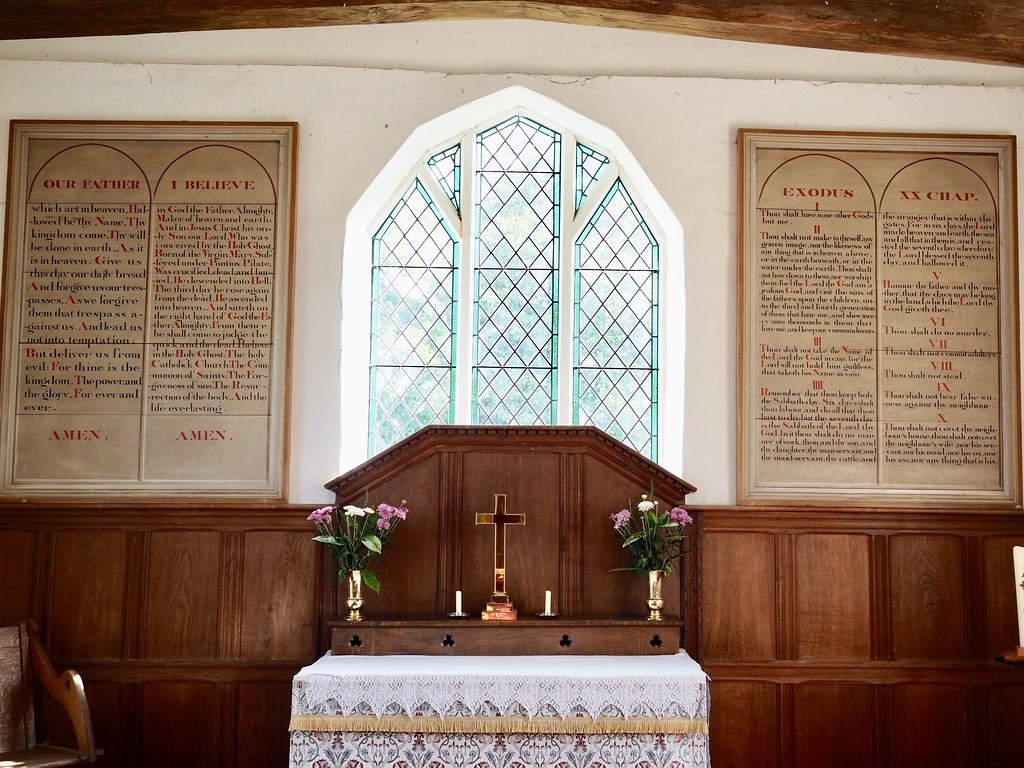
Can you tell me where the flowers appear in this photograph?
[307,489,409,594]
[606,478,695,582]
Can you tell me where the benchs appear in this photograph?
[0,619,105,768]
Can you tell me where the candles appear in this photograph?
[545,590,551,614]
[456,590,461,614]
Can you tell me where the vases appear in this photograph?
[644,570,666,621]
[344,569,367,622]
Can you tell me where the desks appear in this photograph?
[288,649,712,768]
